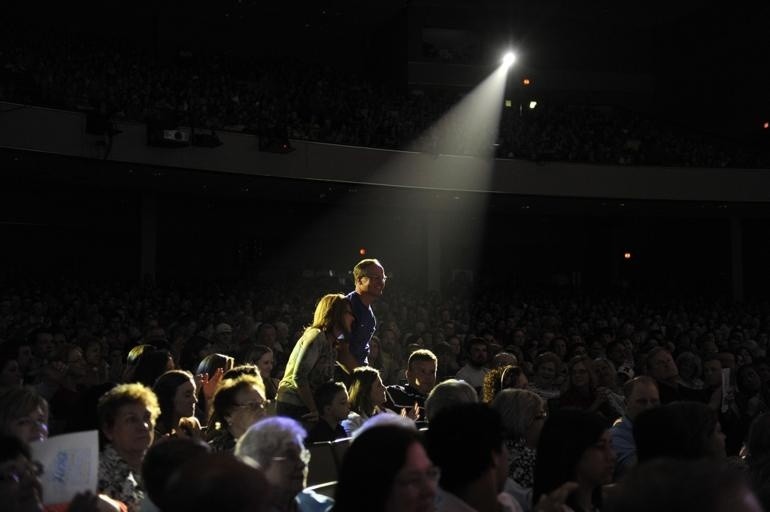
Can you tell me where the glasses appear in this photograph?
[387,467,443,494]
[268,448,311,463]
[362,275,388,285]
[233,400,272,411]
[534,407,549,420]
[1,461,44,489]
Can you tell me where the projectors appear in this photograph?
[150,128,192,148]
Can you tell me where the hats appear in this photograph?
[617,365,635,379]
[123,343,155,376]
[216,323,232,334]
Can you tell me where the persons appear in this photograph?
[1,259,770,511]
[2,37,769,170]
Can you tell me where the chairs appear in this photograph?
[306,481,338,500]
[304,441,342,488]
[334,438,350,469]
